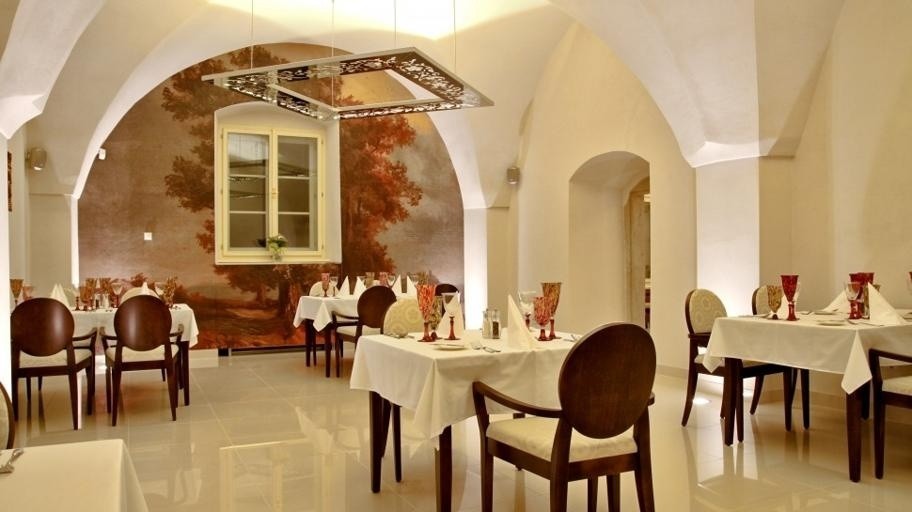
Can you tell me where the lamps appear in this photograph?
[199,1,494,123]
[25,146,48,172]
[506,165,521,185]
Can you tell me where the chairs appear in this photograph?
[9,297,96,431]
[682,289,795,443]
[470,324,657,512]
[868,347,912,479]
[381,298,422,484]
[0,381,15,449]
[330,286,396,378]
[98,295,184,428]
[750,284,810,430]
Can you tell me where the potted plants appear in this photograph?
[267,234,286,258]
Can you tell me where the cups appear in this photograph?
[843,281,862,320]
[429,296,442,339]
[9,278,23,306]
[98,276,110,294]
[780,273,799,322]
[532,295,552,341]
[22,283,32,301]
[519,290,536,330]
[378,271,389,287]
[441,292,461,340]
[862,284,880,319]
[415,282,437,343]
[329,276,337,297]
[765,283,783,320]
[319,271,329,297]
[84,277,97,293]
[110,282,122,308]
[153,282,168,299]
[541,281,562,340]
[364,271,375,289]
[848,272,874,314]
[78,285,92,311]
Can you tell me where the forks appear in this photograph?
[846,318,883,327]
[472,342,500,353]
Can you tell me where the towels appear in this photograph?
[767,285,800,321]
[503,295,545,350]
[406,272,422,297]
[430,292,466,338]
[336,276,351,299]
[866,282,907,324]
[353,277,366,296]
[49,283,69,307]
[820,290,849,312]
[393,274,402,294]
[140,282,150,296]
